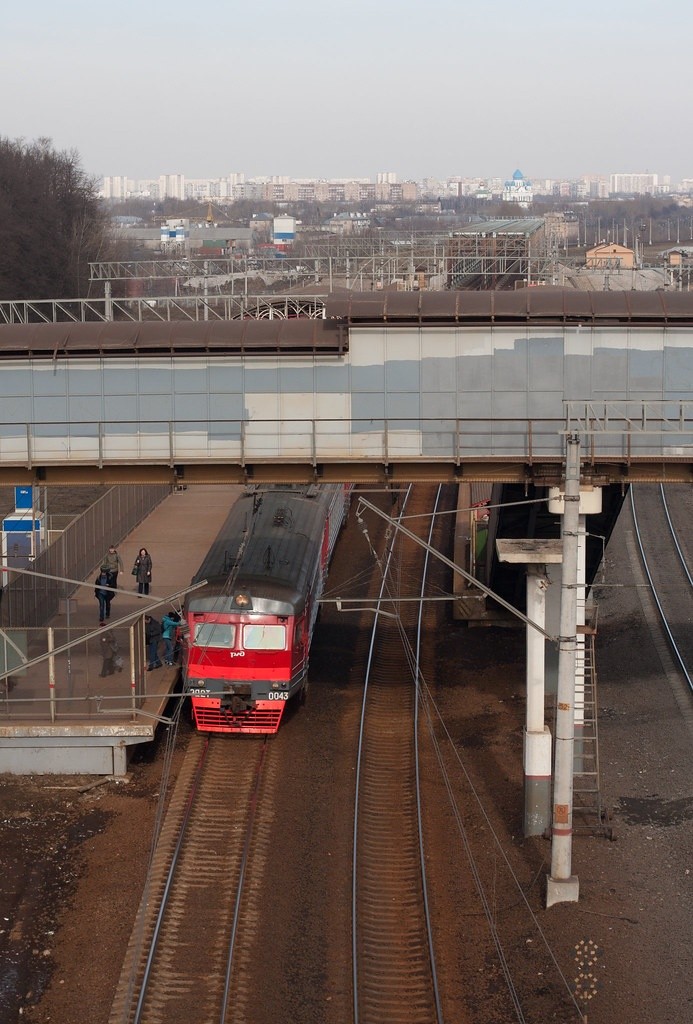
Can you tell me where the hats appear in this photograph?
[100,564,109,572]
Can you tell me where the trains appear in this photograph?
[180,483,353,738]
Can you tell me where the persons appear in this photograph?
[95,565,117,621]
[161,604,185,666]
[145,614,162,672]
[98,623,117,679]
[135,547,152,598]
[103,545,124,587]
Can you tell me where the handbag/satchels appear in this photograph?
[131,564,138,575]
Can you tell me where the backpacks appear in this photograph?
[161,619,170,633]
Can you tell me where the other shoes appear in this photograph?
[106,614,109,618]
[165,659,169,663]
[147,667,153,671]
[100,617,104,621]
[154,663,162,668]
[168,661,175,666]
